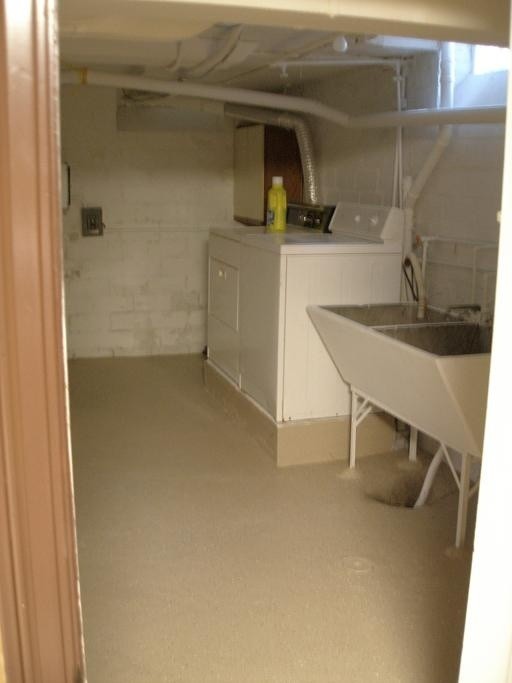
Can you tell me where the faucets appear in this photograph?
[441,302,481,325]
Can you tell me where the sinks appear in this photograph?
[370,323,491,359]
[302,301,463,328]
